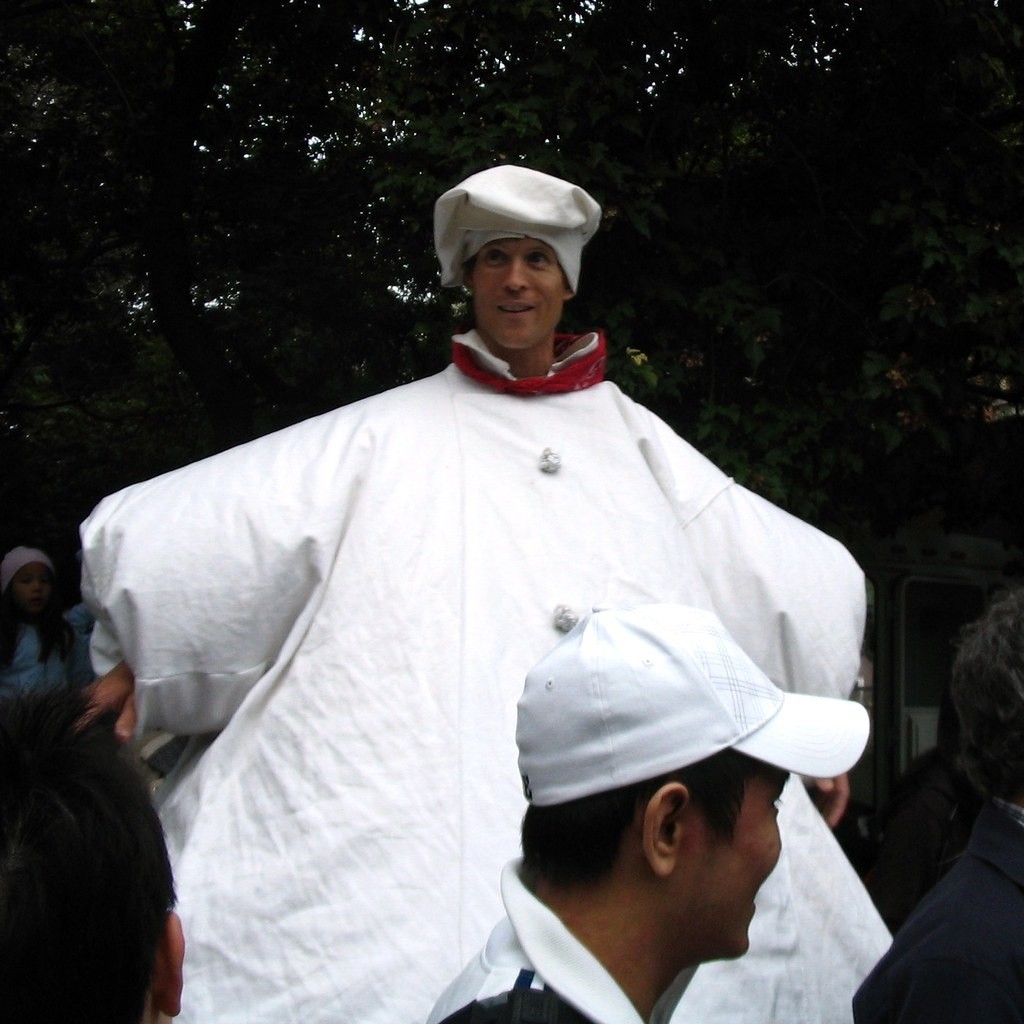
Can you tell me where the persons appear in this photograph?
[851,589,1024,1024]
[426,604,871,1024]
[67,165,895,1024]
[0,545,183,1024]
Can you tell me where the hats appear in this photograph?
[514,601,873,807]
[432,164,603,296]
[0,545,56,597]
